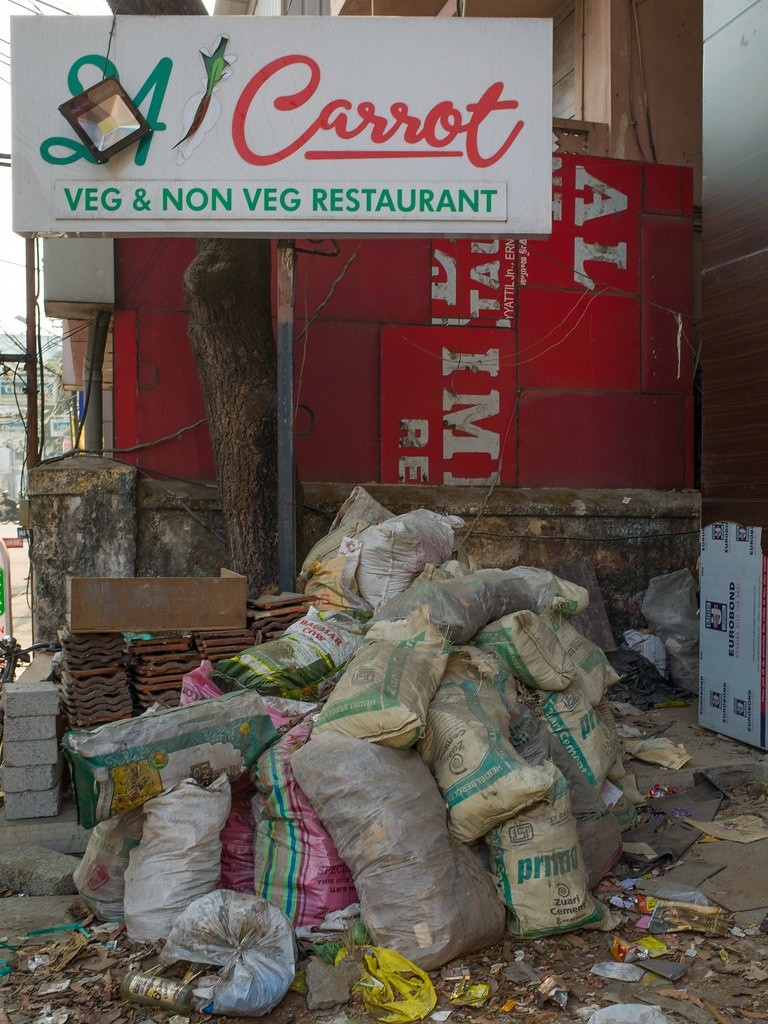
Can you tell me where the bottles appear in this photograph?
[119,970,213,1013]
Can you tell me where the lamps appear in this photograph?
[57,77,151,163]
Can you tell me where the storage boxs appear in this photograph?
[66,567,248,634]
